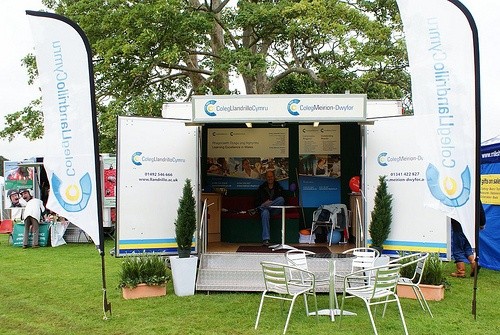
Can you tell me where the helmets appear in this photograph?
[7,189,19,197]
[18,188,31,194]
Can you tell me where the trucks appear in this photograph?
[0,152,116,243]
[109,90,452,293]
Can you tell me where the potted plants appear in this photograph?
[396,250,452,302]
[167,178,199,298]
[119,250,171,301]
[366,176,394,287]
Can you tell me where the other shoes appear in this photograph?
[23,245,29,249]
[248,208,259,217]
[32,245,40,248]
[261,240,269,247]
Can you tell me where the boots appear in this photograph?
[451,262,465,277]
[468,255,481,277]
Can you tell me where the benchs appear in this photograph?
[220,194,300,220]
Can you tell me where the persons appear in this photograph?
[248,170,288,247]
[242,155,340,179]
[7,189,33,208]
[22,197,45,249]
[451,199,486,278]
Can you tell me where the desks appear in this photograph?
[13,222,52,246]
[349,192,365,240]
[202,193,221,244]
[307,251,358,321]
[48,221,90,247]
[266,205,301,252]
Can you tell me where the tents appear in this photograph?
[451,135,500,271]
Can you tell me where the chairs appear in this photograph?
[309,204,351,247]
[254,247,434,335]
[0,220,13,248]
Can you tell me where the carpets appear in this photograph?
[236,246,332,254]
